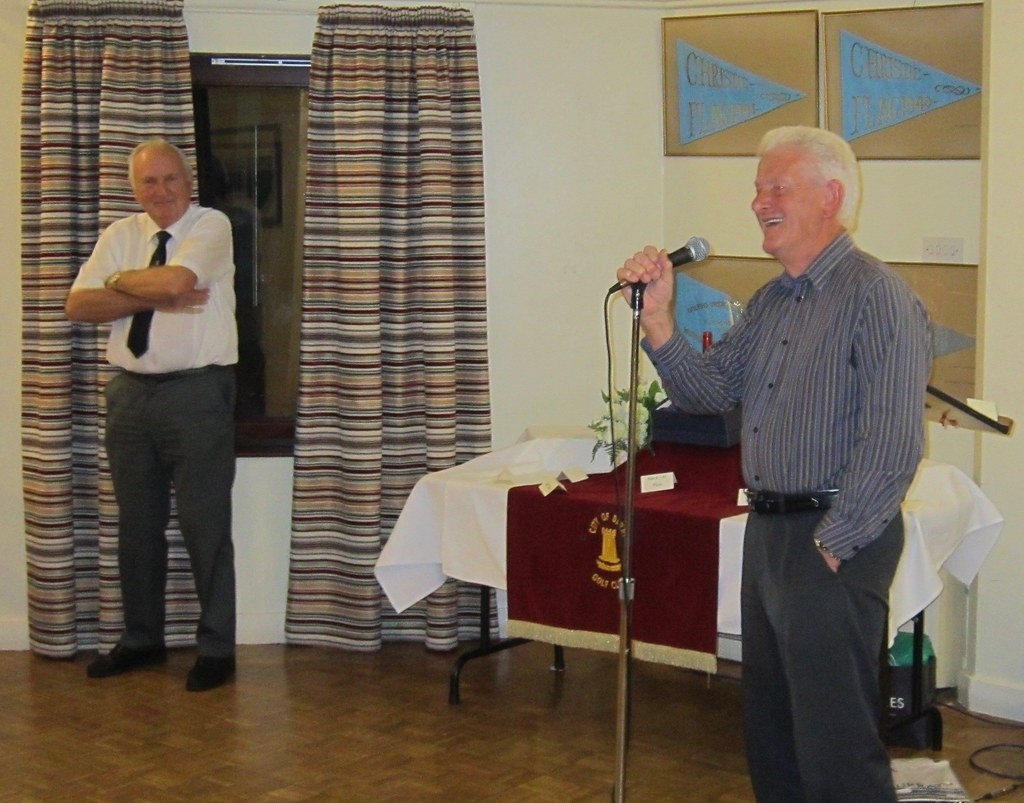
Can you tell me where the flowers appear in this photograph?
[594,382,664,462]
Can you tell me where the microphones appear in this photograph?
[609,236,710,294]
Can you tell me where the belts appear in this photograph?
[122,365,232,382]
[743,490,840,514]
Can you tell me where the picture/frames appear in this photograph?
[660,9,820,158]
[820,2,985,161]
[666,253,786,357]
[883,259,979,429]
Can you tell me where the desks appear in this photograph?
[375,435,1005,757]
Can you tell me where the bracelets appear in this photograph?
[814,539,841,564]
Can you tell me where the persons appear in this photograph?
[65,139,240,693]
[616,127,934,802]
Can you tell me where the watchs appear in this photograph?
[108,270,123,290]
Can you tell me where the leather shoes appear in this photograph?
[186,655,237,691]
[89,641,166,678]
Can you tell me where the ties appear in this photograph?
[128,231,172,360]
